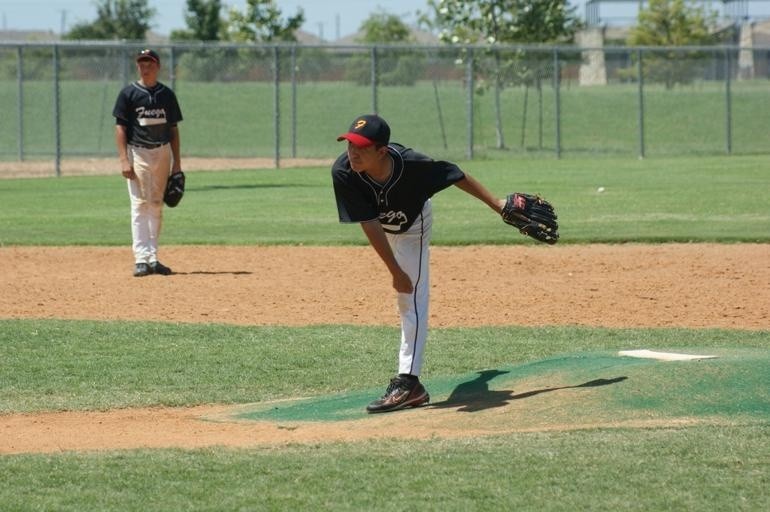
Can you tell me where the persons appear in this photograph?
[331,115,558,414]
[112,48,185,277]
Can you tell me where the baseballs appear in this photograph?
[597,187,606,194]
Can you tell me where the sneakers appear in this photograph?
[149,261,171,275]
[366,380,432,413]
[133,262,148,277]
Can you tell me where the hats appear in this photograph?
[136,49,158,63]
[338,113,391,147]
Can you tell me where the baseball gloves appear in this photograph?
[164,171,185,207]
[500,192,561,244]
